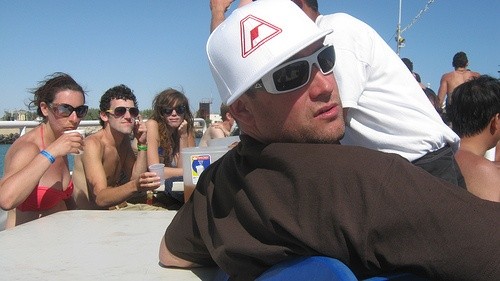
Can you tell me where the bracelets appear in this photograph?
[137,145,147,152]
[40,149,55,164]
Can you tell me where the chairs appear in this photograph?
[254,255,392,281]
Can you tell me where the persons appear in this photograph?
[209,0,465,187]
[402,52,480,114]
[446,75,500,201]
[159,0,500,281]
[72,85,161,210]
[200,103,236,147]
[1,72,89,231]
[145,87,196,178]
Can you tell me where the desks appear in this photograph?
[0,210,231,281]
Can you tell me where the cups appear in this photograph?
[63,130,86,156]
[148,163,166,185]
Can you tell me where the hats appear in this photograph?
[205,0,335,106]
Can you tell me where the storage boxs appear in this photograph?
[206,135,242,146]
[179,144,234,204]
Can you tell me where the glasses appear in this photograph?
[47,101,88,119]
[248,43,337,94]
[164,109,184,117]
[104,106,139,118]
